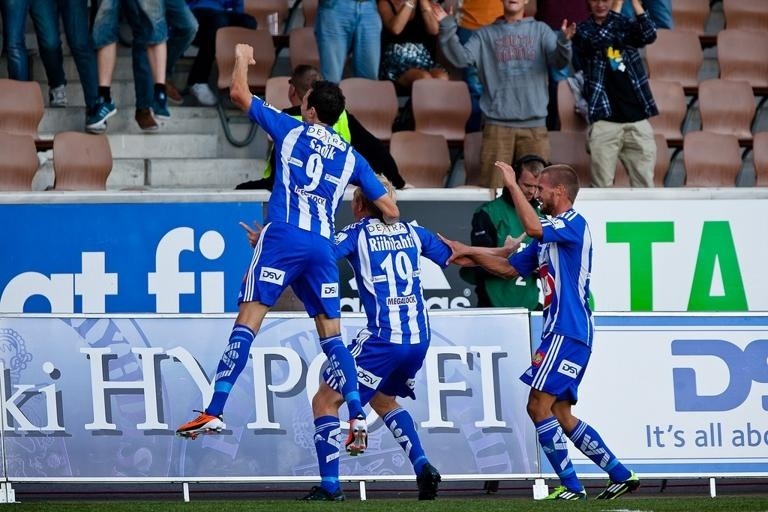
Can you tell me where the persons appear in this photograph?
[176,41,401,458]
[237,184,528,503]
[437,159,643,500]
[461,154,546,311]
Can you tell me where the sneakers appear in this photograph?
[417,463,441,500]
[345,413,368,456]
[545,485,587,501]
[49,85,68,107]
[189,83,217,106]
[176,410,223,440]
[594,470,640,500]
[303,486,346,501]
[136,83,184,130]
[85,97,118,134]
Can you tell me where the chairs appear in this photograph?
[215,1,768,193]
[2,76,113,192]
